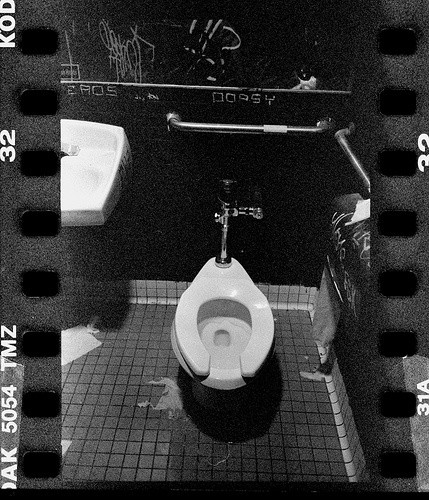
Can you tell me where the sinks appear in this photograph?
[60,118,134,227]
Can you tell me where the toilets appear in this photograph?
[170,254,276,416]
